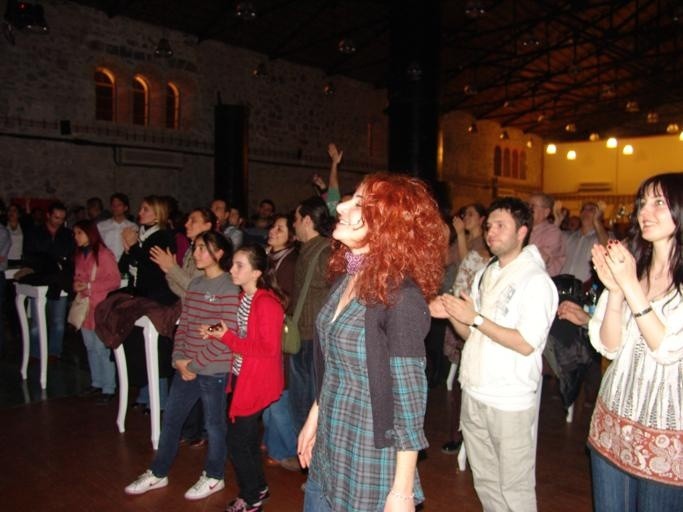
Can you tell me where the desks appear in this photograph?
[12,278,131,390]
[101,308,180,452]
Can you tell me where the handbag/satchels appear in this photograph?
[282,323,302,354]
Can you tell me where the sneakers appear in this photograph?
[184,476,225,500]
[124,469,168,495]
[227,485,270,512]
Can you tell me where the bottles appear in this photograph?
[586,283,599,315]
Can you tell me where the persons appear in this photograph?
[116,145,683,510]
[0,191,138,408]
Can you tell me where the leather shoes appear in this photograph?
[130,402,162,415]
[441,440,460,454]
[81,384,116,406]
[178,437,207,448]
